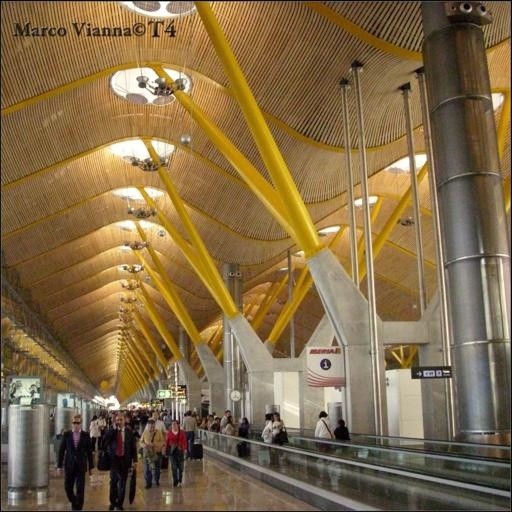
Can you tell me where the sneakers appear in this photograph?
[109,503,124,510]
[146,481,159,489]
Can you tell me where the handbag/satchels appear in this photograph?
[129,468,136,503]
[279,431,288,443]
[98,450,111,470]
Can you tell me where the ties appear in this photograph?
[117,431,122,456]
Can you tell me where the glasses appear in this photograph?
[73,422,80,424]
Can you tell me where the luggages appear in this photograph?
[162,454,168,469]
[192,444,203,459]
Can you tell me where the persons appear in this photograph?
[88,407,250,510]
[334,419,350,440]
[261,412,287,468]
[56,414,95,511]
[314,411,335,465]
